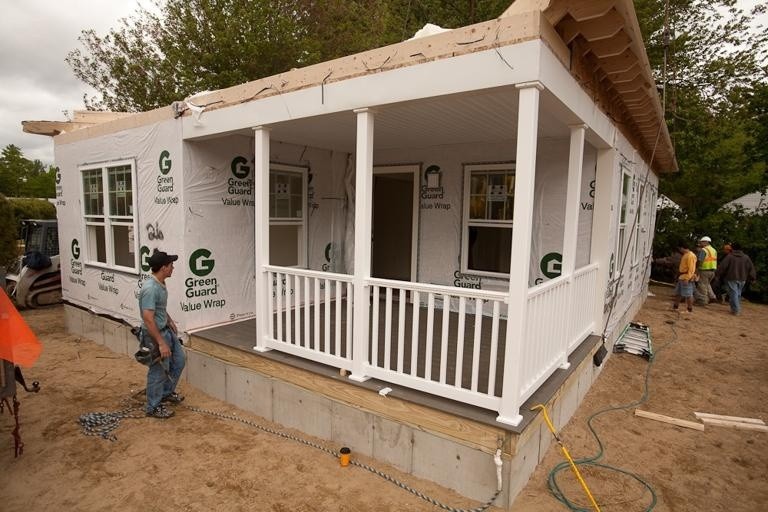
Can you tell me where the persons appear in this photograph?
[138,252,185,418]
[717,239,756,317]
[670,242,697,316]
[696,235,718,307]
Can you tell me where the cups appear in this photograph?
[340,447,351,467]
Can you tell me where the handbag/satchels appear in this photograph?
[135,351,152,366]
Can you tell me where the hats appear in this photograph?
[150,252,178,268]
[700,236,711,242]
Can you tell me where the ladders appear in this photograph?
[613,321,652,362]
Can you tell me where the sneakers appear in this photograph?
[145,405,175,419]
[161,392,185,403]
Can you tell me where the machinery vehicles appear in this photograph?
[6,219,62,309]
